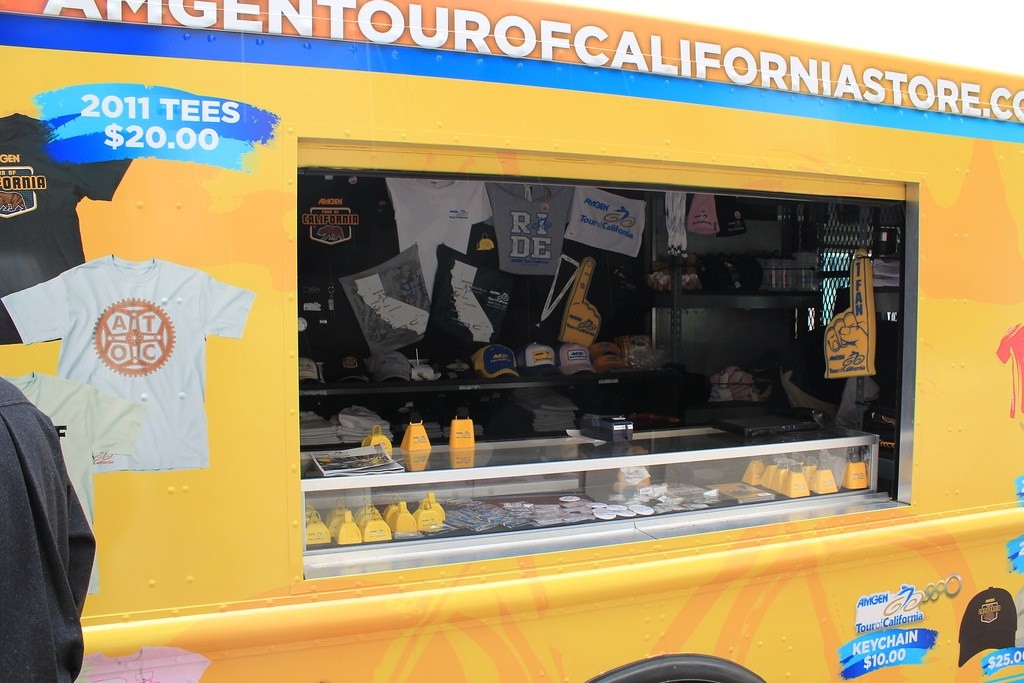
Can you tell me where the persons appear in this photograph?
[0,378,96,683]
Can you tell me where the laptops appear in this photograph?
[713,414,818,436]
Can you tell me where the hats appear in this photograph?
[300,355,326,389]
[473,344,520,378]
[336,355,370,383]
[589,342,623,373]
[560,344,595,376]
[518,341,561,376]
[373,351,411,383]
